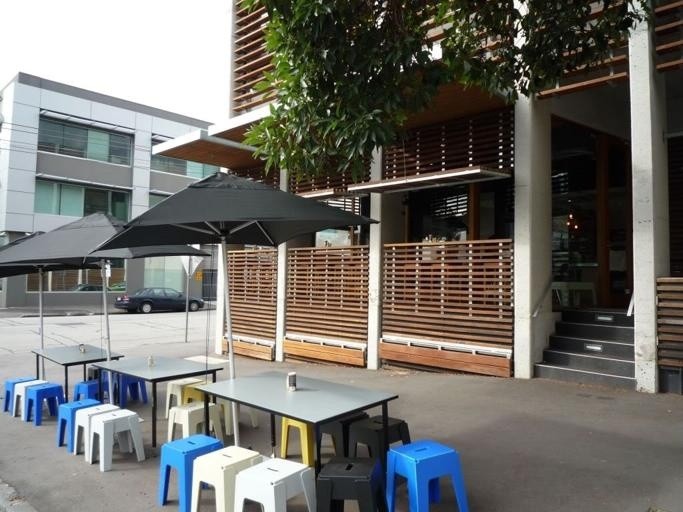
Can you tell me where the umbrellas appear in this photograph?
[0,231,102,381]
[0,211,212,404]
[87,171,382,447]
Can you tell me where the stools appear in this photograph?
[4,366,148,471]
[306,260,510,311]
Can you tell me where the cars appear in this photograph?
[71,284,110,292]
[109,282,126,291]
[115,286,204,312]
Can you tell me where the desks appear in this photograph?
[196,370,399,488]
[92,357,223,448]
[31,344,125,403]
[552,281,598,307]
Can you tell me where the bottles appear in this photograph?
[147,355,153,368]
[78,343,84,352]
[286,372,296,392]
